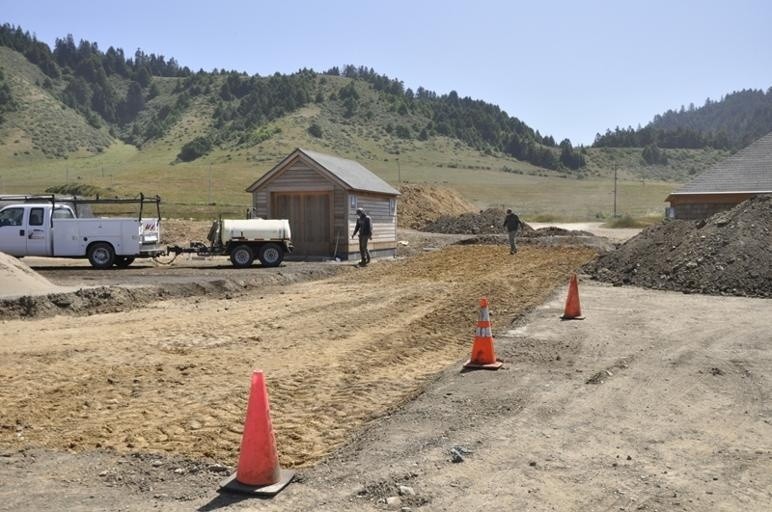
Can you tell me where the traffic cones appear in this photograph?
[213,365,300,498]
[556,273,587,321]
[459,295,504,369]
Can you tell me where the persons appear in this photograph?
[351,208,373,265]
[503,208,523,254]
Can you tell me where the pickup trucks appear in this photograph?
[0,193,165,269]
